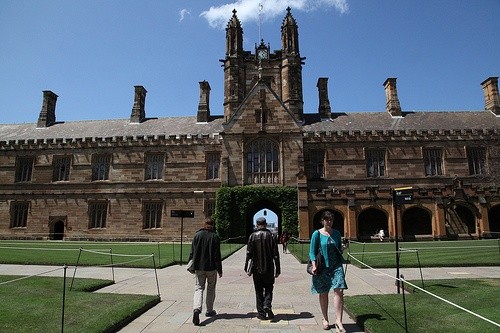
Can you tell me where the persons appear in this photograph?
[309,211,348,333]
[244,218,281,319]
[189,219,223,326]
[276,232,279,243]
[281,232,289,252]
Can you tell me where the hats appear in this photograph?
[256,216,267,225]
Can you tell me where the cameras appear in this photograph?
[343,239,348,244]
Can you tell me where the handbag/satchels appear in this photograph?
[247,260,254,276]
[307,256,319,275]
[186,258,195,274]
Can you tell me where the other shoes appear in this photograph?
[256,311,265,319]
[335,323,346,333]
[192,309,201,326]
[263,306,276,319]
[321,318,330,330]
[205,309,216,317]
